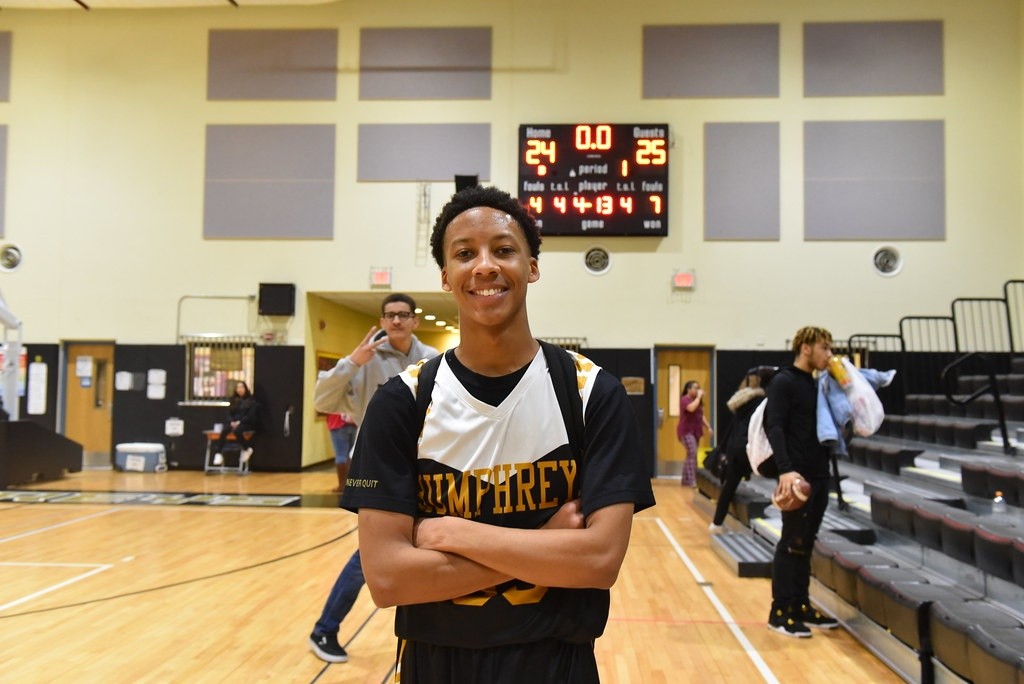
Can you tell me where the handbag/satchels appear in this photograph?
[841,357,885,436]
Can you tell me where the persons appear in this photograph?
[711,367,780,528]
[340,188,657,684]
[214,382,254,464]
[768,326,845,638]
[328,414,357,492]
[677,381,713,490]
[309,294,438,662]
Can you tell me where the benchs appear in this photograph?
[203,429,255,473]
[697,359,1024,684]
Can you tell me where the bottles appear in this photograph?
[27,356,48,414]
[828,357,853,391]
[992,490,1007,514]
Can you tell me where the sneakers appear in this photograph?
[769,606,814,636]
[309,632,349,662]
[800,598,840,629]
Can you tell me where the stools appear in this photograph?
[116,442,165,471]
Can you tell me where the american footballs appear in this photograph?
[770,478,813,513]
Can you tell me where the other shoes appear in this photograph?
[709,521,732,535]
[213,453,224,464]
[241,446,253,462]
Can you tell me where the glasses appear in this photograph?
[384,311,413,321]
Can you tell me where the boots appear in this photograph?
[332,463,348,491]
